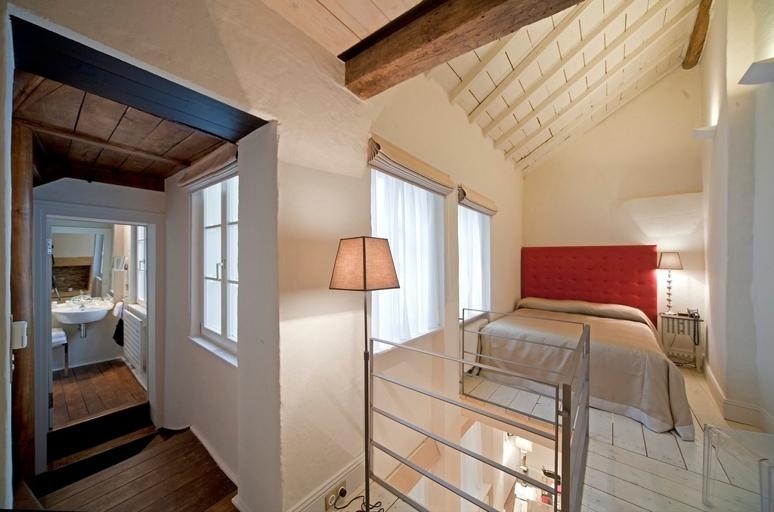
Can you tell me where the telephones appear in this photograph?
[687,308,700,318]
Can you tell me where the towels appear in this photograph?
[111,300,123,345]
[49,327,68,347]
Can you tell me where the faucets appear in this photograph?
[78,293,84,311]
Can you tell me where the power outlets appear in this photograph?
[324,478,347,512]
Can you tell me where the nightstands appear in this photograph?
[659,309,705,374]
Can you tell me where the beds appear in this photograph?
[475,243,662,431]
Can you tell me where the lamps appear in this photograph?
[328,235,402,511]
[656,250,685,316]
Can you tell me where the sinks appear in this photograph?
[52,303,109,323]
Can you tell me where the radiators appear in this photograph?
[123,310,145,373]
